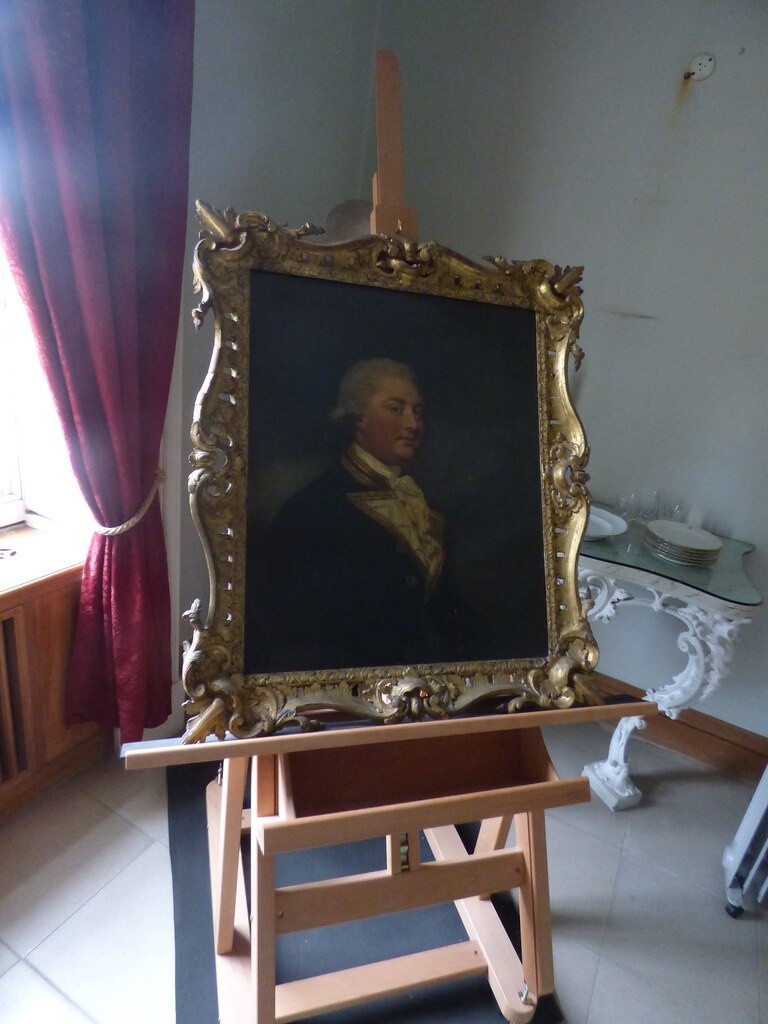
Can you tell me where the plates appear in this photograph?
[643,520,723,567]
[582,505,628,542]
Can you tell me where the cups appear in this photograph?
[615,490,682,527]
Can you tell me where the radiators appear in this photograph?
[720,765,768,918]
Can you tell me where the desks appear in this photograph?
[574,502,761,811]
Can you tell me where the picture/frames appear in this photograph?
[179,198,607,747]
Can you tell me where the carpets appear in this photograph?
[162,759,566,1024]
